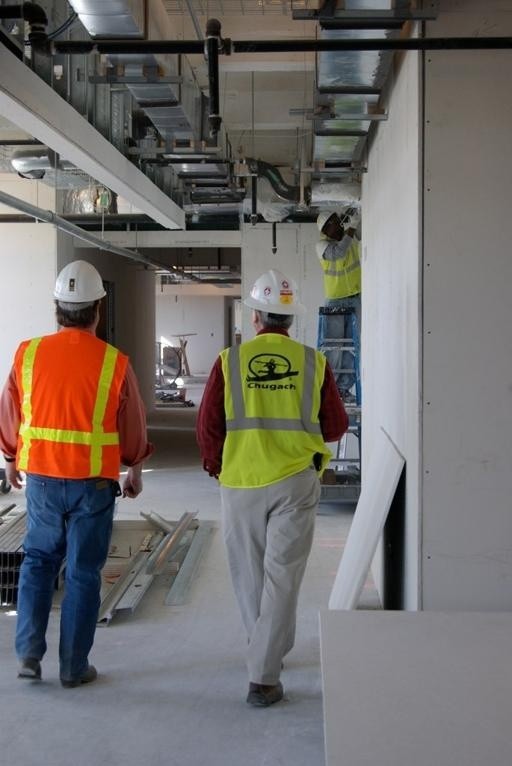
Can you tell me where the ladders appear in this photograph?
[317,306,362,505]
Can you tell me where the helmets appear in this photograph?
[52,258,108,304]
[243,269,306,316]
[316,211,336,232]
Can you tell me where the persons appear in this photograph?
[314,207,362,403]
[1,259,157,690]
[196,267,350,707]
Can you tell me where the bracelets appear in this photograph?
[3,453,17,464]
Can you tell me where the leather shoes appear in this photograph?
[60,665,98,689]
[16,656,43,681]
[246,664,284,707]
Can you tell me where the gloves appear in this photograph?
[347,210,361,230]
